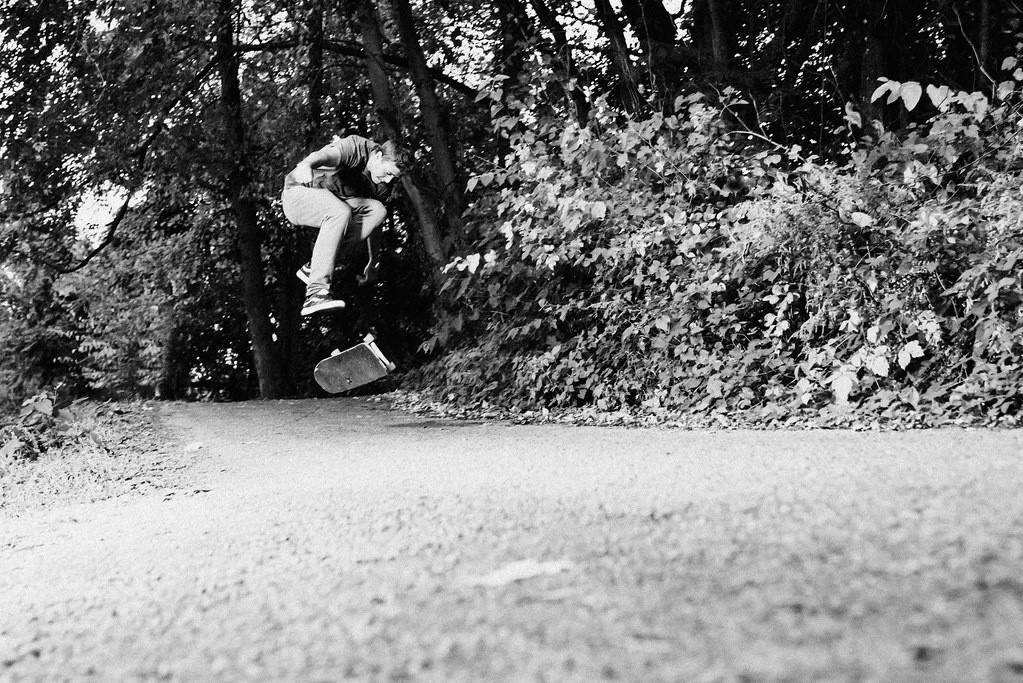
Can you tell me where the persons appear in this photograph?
[276,134,409,317]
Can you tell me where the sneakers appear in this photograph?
[300,288,346,316]
[296,261,311,286]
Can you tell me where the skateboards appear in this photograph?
[313,332,397,393]
